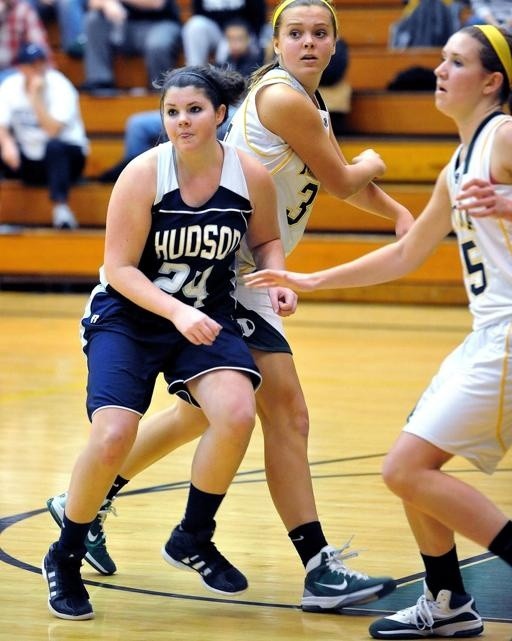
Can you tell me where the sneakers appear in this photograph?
[42,543,93,621]
[52,203,78,232]
[47,487,117,575]
[299,534,396,610]
[161,521,248,596]
[368,577,485,640]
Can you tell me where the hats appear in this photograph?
[12,42,44,63]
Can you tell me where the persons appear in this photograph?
[389,1,512,49]
[1,0,352,231]
[44,0,415,617]
[38,62,302,621]
[242,22,511,641]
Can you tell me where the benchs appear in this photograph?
[0,0,510,308]
